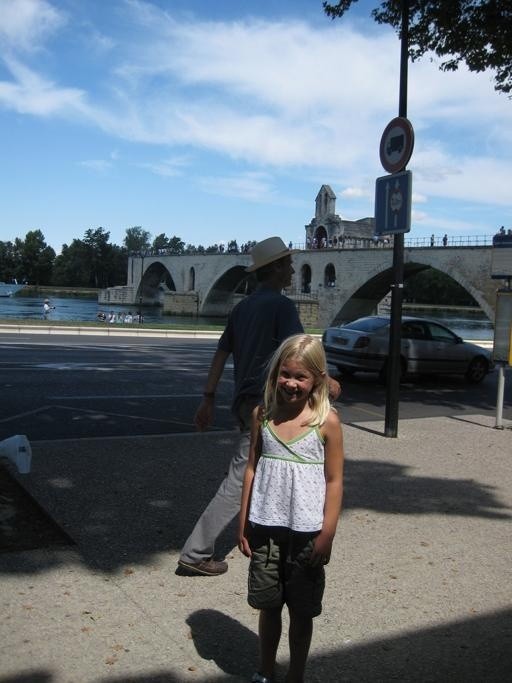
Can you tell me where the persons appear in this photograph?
[372,235,391,246]
[431,234,434,248]
[307,236,345,249]
[97,311,144,324]
[289,241,292,248]
[500,226,512,236]
[43,299,55,321]
[131,241,251,256]
[238,334,344,683]
[178,237,342,576]
[443,234,448,247]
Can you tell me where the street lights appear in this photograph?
[139,245,147,324]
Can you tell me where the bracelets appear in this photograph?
[203,392,215,399]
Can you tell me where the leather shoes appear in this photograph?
[177,556,228,576]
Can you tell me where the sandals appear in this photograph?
[252,672,273,682]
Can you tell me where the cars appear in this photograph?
[322,315,495,385]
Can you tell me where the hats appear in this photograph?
[245,237,302,274]
